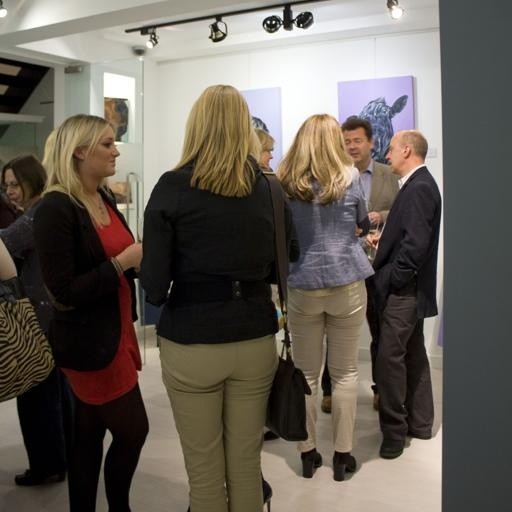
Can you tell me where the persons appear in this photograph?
[251,129,290,442]
[317,115,382,414]
[32,114,149,511]
[367,129,438,459]
[276,114,371,481]
[4,155,68,486]
[141,84,299,511]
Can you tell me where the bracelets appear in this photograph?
[110,256,124,277]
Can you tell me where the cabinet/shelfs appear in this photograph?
[64,64,145,347]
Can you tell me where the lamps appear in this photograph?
[384,1,403,19]
[146,29,159,49]
[261,4,314,33]
[207,17,228,42]
[0,0,7,18]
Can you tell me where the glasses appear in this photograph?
[3,181,20,190]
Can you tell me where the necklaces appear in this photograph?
[78,186,108,223]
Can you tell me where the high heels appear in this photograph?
[301,447,322,477]
[261,474,274,511]
[332,450,357,481]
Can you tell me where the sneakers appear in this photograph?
[320,394,434,459]
[14,470,66,486]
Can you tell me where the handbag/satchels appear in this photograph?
[267,341,312,442]
[1,282,55,403]
